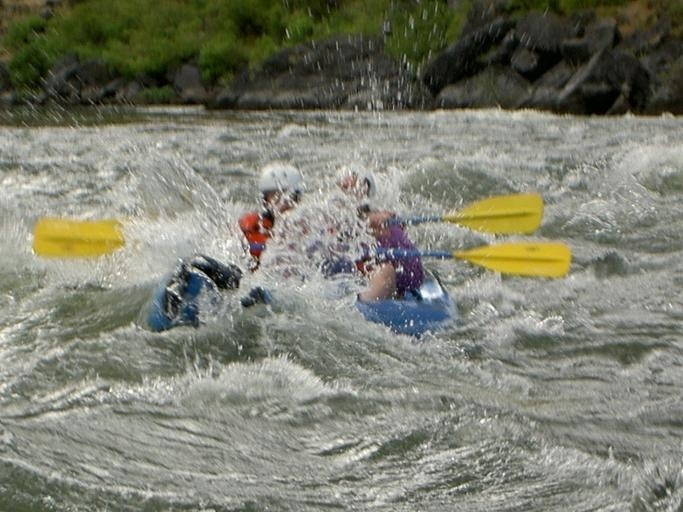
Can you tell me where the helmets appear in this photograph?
[330,163,377,212]
[258,163,304,213]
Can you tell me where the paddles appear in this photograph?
[379,194,542,235]
[33,218,266,260]
[358,243,570,277]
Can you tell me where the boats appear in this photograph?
[149,215,460,344]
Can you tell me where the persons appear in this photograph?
[222,161,426,304]
